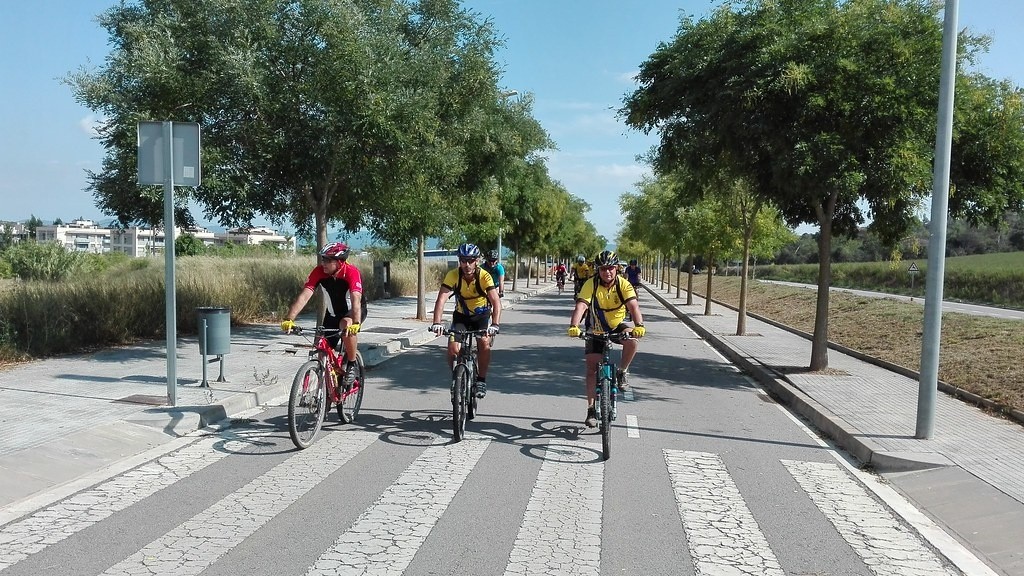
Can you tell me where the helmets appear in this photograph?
[578,256,586,262]
[630,260,637,265]
[596,251,619,269]
[457,244,480,257]
[319,242,349,260]
[487,250,499,259]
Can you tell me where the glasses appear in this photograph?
[600,266,615,271]
[459,257,476,263]
[321,258,336,263]
[487,258,496,262]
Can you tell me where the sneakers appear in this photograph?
[617,368,629,392]
[475,382,486,397]
[585,407,596,427]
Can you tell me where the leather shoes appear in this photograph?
[343,365,358,384]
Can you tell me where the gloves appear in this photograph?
[567,327,581,337]
[631,327,645,338]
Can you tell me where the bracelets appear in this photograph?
[499,291,503,293]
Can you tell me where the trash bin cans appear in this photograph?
[196,307,231,355]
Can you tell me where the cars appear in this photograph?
[616,262,628,274]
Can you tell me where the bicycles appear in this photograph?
[554,274,568,296]
[574,330,647,460]
[488,294,505,348]
[284,326,366,449]
[427,327,500,443]
[625,285,643,321]
[568,278,589,302]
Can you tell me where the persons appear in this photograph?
[280,242,368,412]
[479,250,504,297]
[554,264,568,286]
[570,256,641,298]
[432,244,501,404]
[568,249,646,427]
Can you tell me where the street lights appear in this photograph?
[495,90,518,264]
